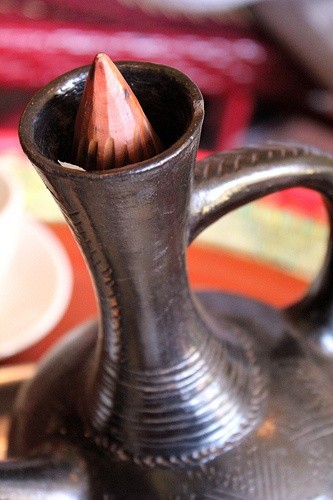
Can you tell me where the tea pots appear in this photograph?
[0,53,333,500]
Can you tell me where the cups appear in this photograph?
[0,171,24,285]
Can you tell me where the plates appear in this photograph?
[0,217,71,360]
